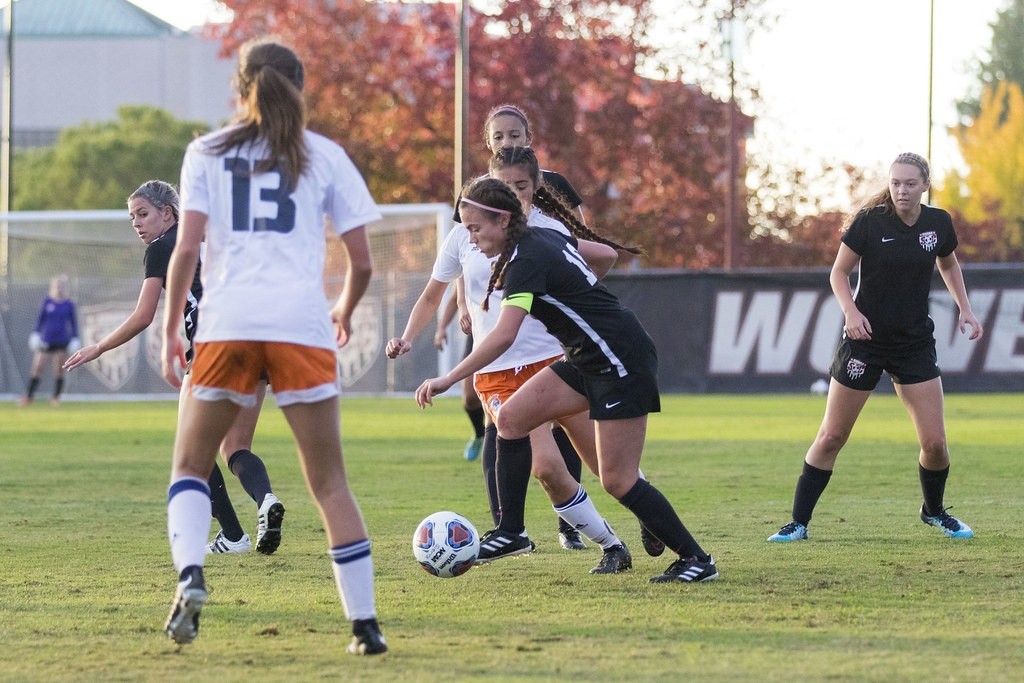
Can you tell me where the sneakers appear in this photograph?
[649,553,718,584]
[558,528,587,550]
[463,434,484,461]
[344,633,388,656]
[639,520,666,557]
[472,526,536,563]
[920,502,974,539]
[163,569,209,644]
[588,541,633,573]
[766,520,808,542]
[255,492,285,556]
[206,529,251,554]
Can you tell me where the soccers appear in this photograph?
[411,509,482,577]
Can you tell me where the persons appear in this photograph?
[450,106,587,551]
[386,145,665,576]
[165,41,388,658]
[61,179,285,555]
[766,152,984,543]
[434,277,487,461]
[23,276,79,407]
[414,178,719,584]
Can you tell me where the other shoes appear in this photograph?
[20,397,29,405]
[50,399,59,406]
